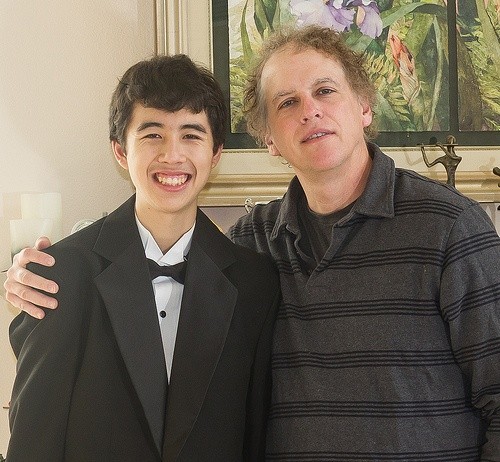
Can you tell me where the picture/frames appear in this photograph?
[153,0,500,208]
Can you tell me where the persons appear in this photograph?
[3,26,500,461]
[5,54,281,462]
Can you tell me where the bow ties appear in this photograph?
[147,258,187,284]
